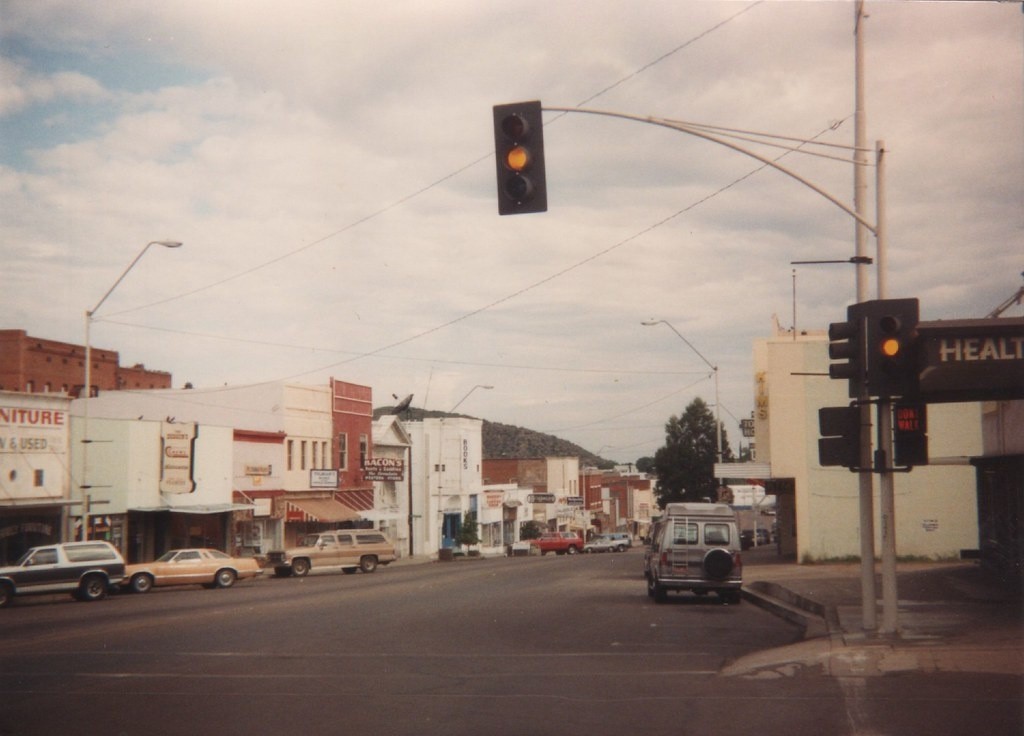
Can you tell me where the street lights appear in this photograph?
[82,237,182,540]
[640,318,727,488]
[438,382,496,551]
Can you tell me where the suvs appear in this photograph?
[649,502,744,602]
[644,520,656,577]
[593,533,632,553]
[264,529,399,579]
[0,539,126,605]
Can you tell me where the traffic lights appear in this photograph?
[893,400,931,466]
[868,297,924,398]
[494,99,548,216]
[828,302,868,400]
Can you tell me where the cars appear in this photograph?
[583,538,618,553]
[521,531,584,555]
[118,548,263,594]
